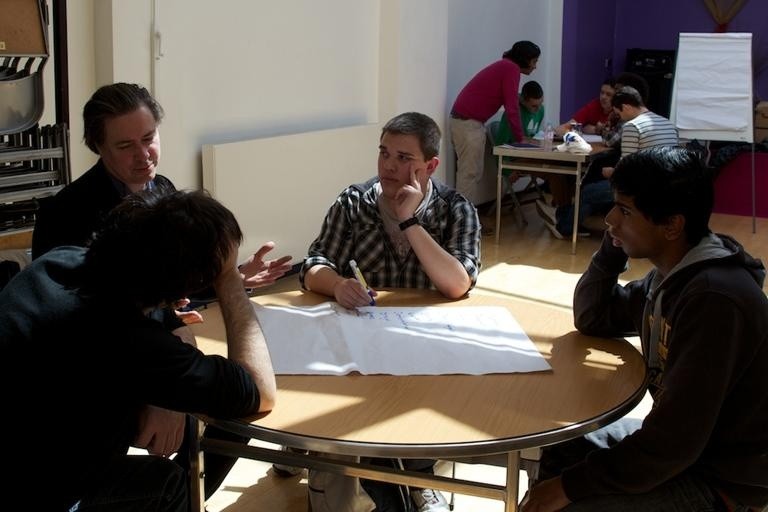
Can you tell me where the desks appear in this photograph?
[172,280,653,510]
[493,129,615,255]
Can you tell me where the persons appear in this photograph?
[31,82,293,500]
[299,112,481,512]
[494,80,572,204]
[0,183,277,512]
[449,41,541,235]
[556,80,622,202]
[534,86,680,242]
[516,145,764,512]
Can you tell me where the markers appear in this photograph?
[348,259,377,306]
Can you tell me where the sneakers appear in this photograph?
[410,488,451,512]
[577,226,592,237]
[272,445,308,477]
[534,198,559,226]
[479,221,495,236]
[541,219,565,240]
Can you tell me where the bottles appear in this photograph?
[570,122,576,133]
[544,121,553,153]
[578,122,583,136]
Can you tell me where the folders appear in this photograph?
[504,142,543,150]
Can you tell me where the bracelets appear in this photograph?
[393,216,421,231]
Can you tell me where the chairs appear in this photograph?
[485,121,544,225]
[1,122,71,253]
[0,0,50,132]
